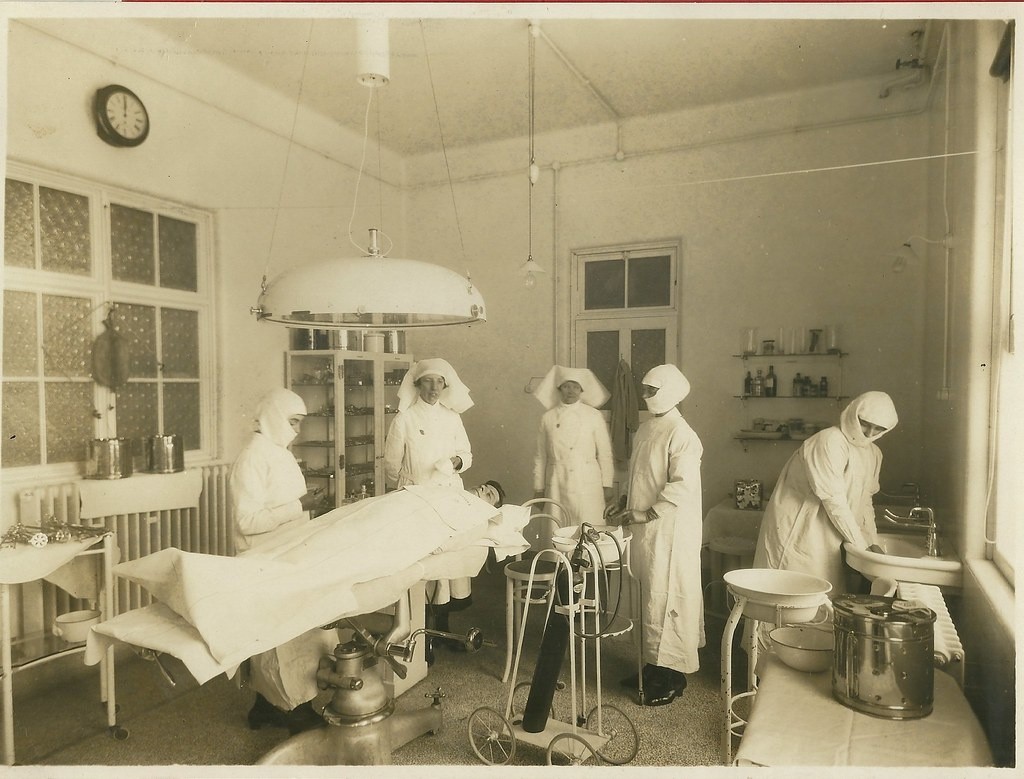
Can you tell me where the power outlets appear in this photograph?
[928,471,950,501]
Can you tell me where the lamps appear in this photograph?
[514,26,544,289]
[250,18,489,332]
[529,34,539,188]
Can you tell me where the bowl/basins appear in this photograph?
[769,627,836,673]
[552,525,634,564]
[722,569,833,624]
[55,610,101,643]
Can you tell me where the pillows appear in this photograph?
[493,504,530,532]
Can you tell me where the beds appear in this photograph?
[83,487,531,766]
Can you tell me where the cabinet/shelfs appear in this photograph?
[0,524,132,766]
[732,352,850,447]
[286,350,415,520]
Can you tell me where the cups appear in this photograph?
[736,323,838,353]
[305,329,317,350]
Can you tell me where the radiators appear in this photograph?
[19,461,242,636]
[896,582,965,697]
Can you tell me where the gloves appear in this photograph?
[532,491,546,511]
[613,507,658,526]
[603,494,628,523]
[603,487,613,502]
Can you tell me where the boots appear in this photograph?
[424,612,458,667]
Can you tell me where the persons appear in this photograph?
[529,364,615,628]
[231,388,341,734]
[604,363,706,704]
[116,479,504,631]
[383,358,475,633]
[739,390,901,656]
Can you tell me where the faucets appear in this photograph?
[878,482,920,507]
[883,506,940,556]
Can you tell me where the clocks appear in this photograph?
[92,85,150,148]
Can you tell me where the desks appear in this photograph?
[733,648,994,769]
[710,497,942,616]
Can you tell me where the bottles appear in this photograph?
[744,365,827,398]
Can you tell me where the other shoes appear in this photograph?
[619,663,687,706]
[287,700,327,736]
[248,693,286,729]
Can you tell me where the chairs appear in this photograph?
[499,497,571,685]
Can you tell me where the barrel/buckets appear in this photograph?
[832,594,938,721]
[150,434,184,474]
[364,334,385,354]
[85,438,132,480]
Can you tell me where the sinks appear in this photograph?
[844,532,963,588]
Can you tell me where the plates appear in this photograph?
[789,434,810,440]
[738,431,782,440]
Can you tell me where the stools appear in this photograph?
[709,537,756,614]
